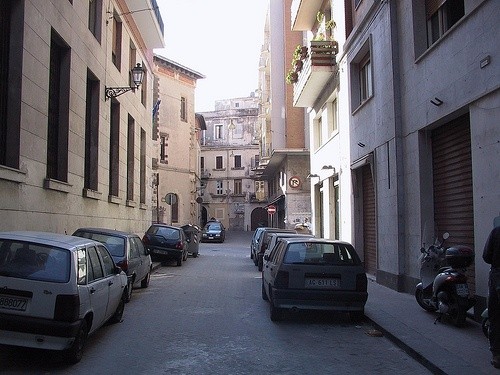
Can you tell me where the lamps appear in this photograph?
[321,165,335,170]
[307,174,319,178]
[105,63,146,102]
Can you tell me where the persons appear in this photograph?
[482,226,500,370]
[303,217,311,233]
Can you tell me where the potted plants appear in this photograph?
[285,45,307,85]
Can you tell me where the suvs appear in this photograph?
[0,229,130,367]
[200,219,226,243]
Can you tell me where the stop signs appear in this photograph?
[267,205,277,215]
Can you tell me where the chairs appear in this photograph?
[12,247,36,272]
[322,252,339,263]
[112,244,125,257]
[284,251,301,262]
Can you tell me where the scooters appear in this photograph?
[414,231,475,326]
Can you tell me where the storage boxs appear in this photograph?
[445,245,475,269]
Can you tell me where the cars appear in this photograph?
[261,237,368,323]
[70,227,153,303]
[142,223,191,266]
[250,226,319,270]
